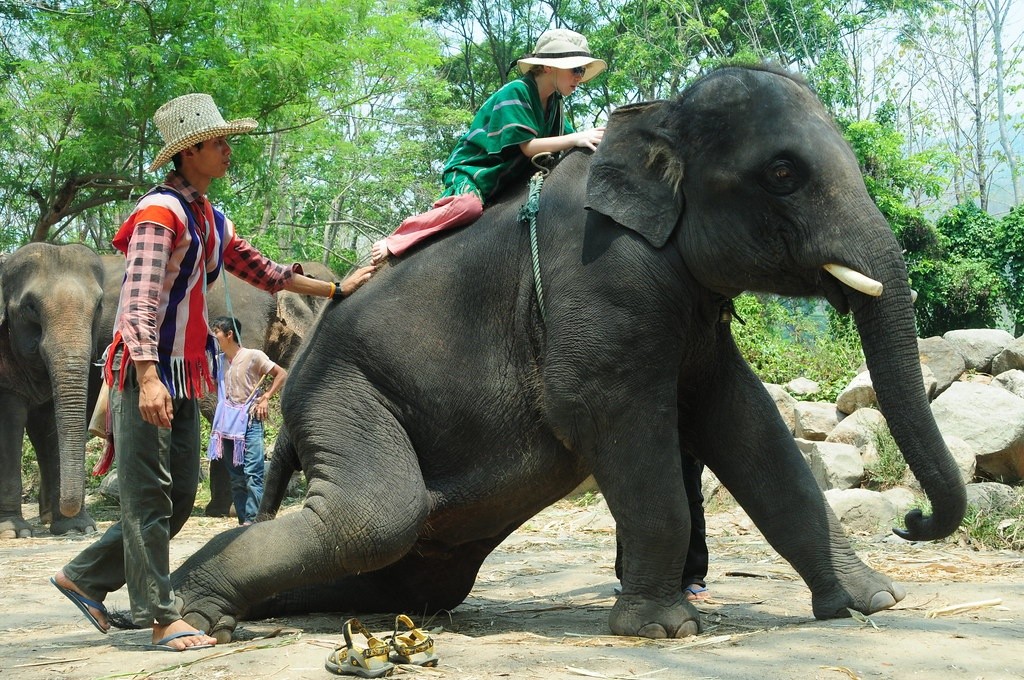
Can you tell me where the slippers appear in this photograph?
[143,629,215,651]
[49,576,111,634]
[614,586,622,594]
[683,586,712,603]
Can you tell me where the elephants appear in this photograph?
[0,61,968,644]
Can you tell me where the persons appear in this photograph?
[371,30,608,266]
[51,93,376,651]
[207,316,288,526]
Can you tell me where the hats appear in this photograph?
[147,93,260,173]
[517,29,607,83]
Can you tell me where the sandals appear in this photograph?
[381,615,438,667]
[325,617,394,679]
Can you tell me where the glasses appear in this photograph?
[572,66,586,77]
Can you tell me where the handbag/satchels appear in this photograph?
[206,401,249,466]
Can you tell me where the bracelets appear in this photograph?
[329,281,343,303]
